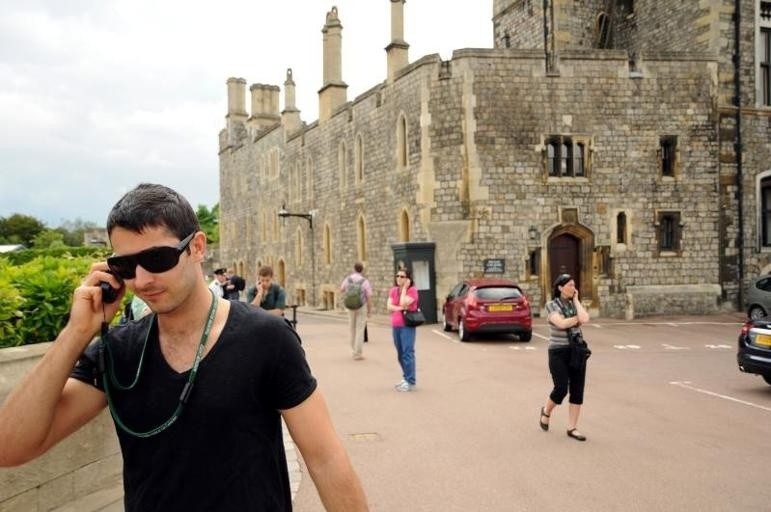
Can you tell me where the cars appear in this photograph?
[737,314,771,385]
[746,273,771,321]
[442,279,533,341]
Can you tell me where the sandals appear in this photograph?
[566,428,587,441]
[540,405,550,430]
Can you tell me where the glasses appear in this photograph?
[106,232,195,279]
[397,274,408,278]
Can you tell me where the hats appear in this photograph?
[215,268,228,276]
[555,274,571,287]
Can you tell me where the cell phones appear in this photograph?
[101,255,122,302]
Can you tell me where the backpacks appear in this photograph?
[237,277,245,290]
[344,277,366,309]
[554,298,591,372]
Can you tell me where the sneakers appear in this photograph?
[354,352,364,362]
[396,380,417,391]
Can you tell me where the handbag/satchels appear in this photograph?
[402,305,426,325]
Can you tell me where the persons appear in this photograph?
[248,267,287,317]
[209,267,227,300]
[0,184,369,511]
[132,291,151,322]
[342,264,372,361]
[539,273,592,441]
[226,269,245,301]
[387,268,425,392]
